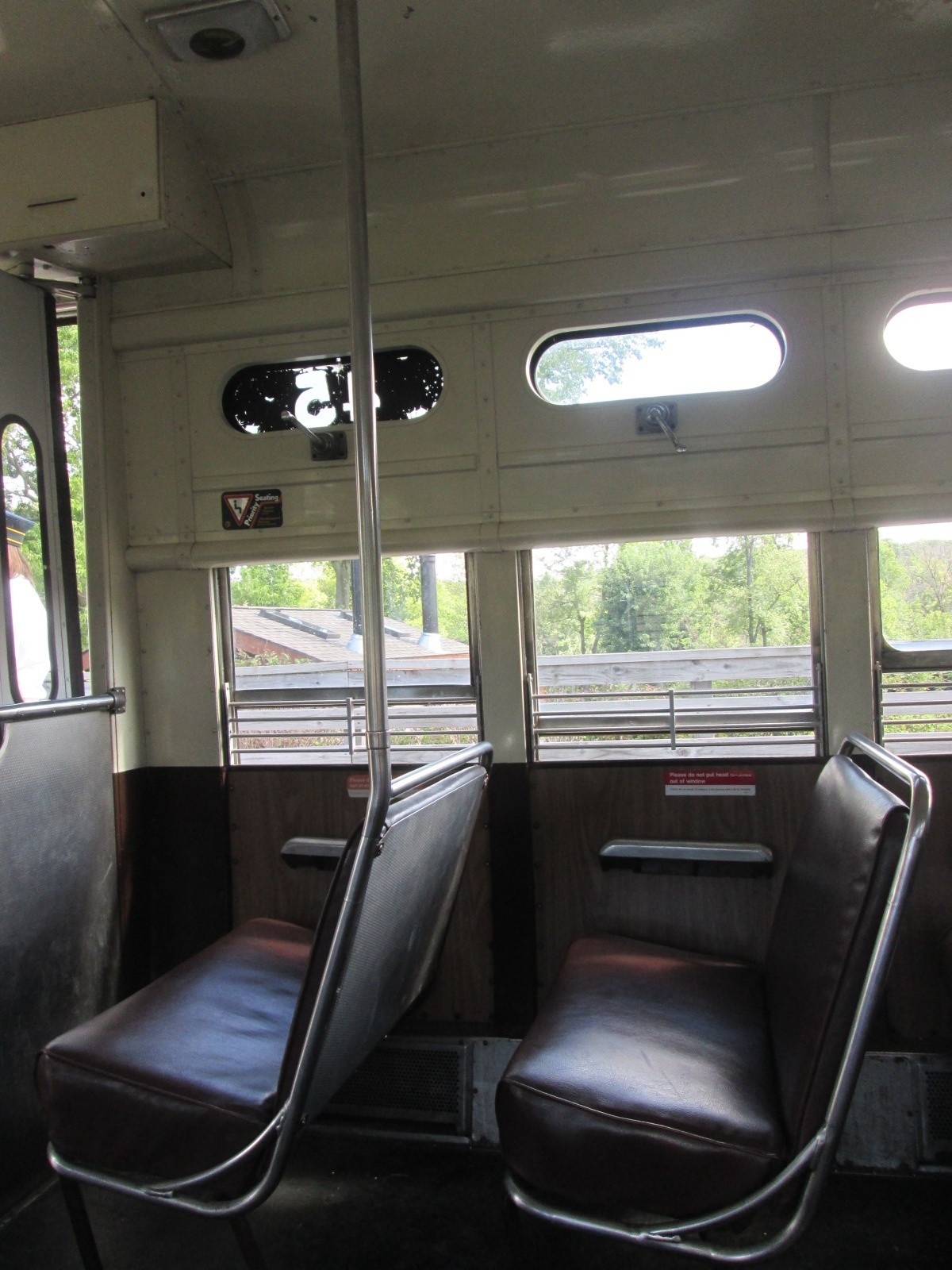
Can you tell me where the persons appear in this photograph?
[6,511,51,703]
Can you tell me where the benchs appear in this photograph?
[490,728,935,1270]
[40,733,510,1263]
